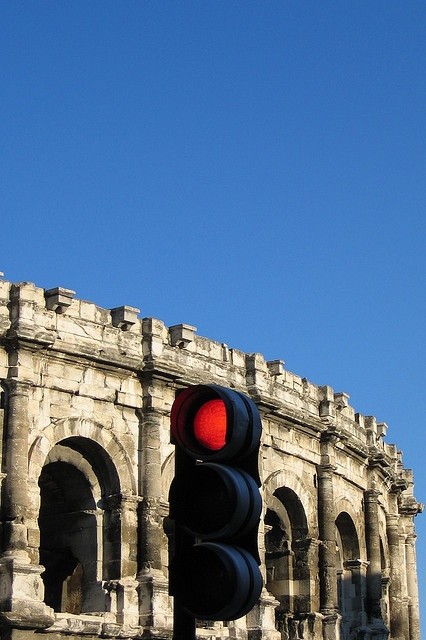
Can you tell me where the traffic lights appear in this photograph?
[169,383,263,626]
